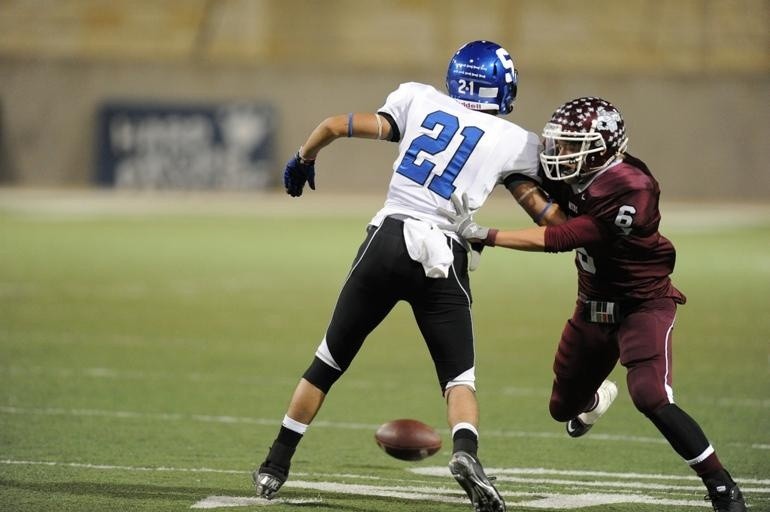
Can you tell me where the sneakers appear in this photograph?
[448,450,506,512]
[703,477,746,512]
[251,471,284,500]
[566,379,618,438]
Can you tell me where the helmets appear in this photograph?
[540,97,629,185]
[445,40,518,116]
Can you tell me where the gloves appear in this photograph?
[436,192,491,272]
[283,151,316,197]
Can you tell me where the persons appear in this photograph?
[248,39,569,512]
[435,95,748,512]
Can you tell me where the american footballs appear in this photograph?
[375,419,442,460]
